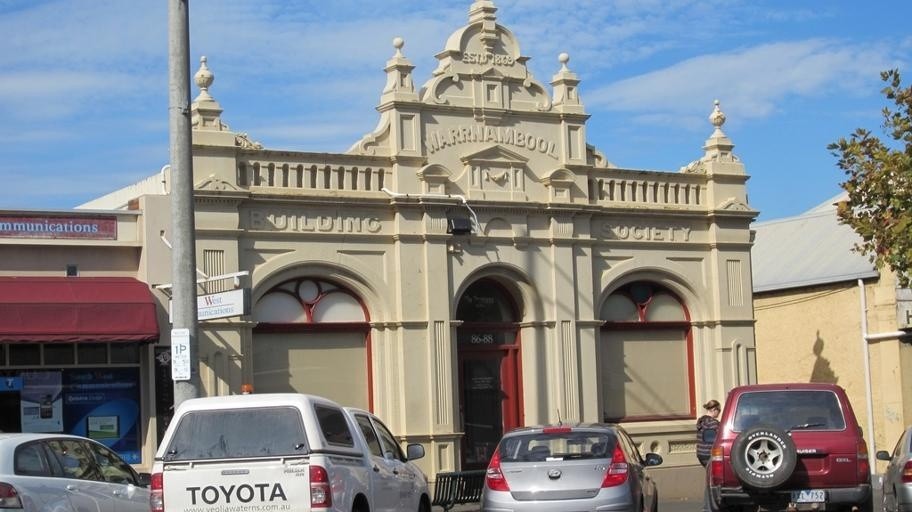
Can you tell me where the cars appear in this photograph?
[477,423,661,511]
[0,429,151,509]
[876,423,912,512]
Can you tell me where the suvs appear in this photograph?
[150,395,432,511]
[705,382,873,511]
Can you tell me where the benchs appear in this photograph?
[431,470,487,512]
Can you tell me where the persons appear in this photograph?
[694,398,722,470]
